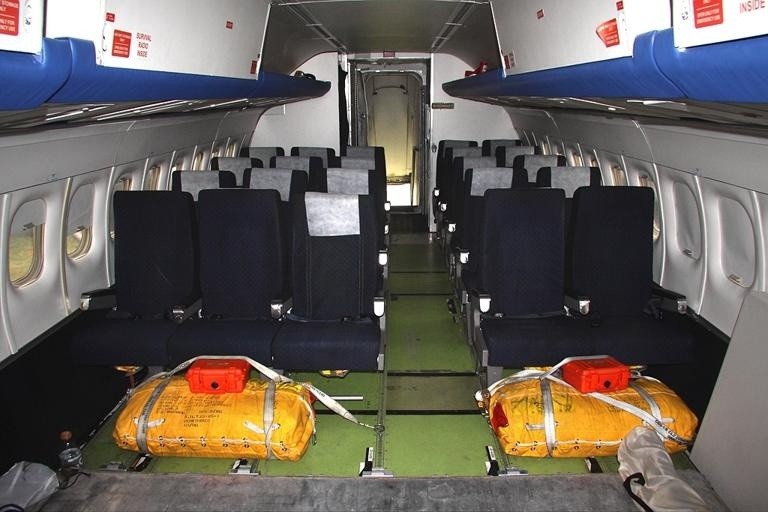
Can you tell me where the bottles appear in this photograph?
[59,431,84,479]
